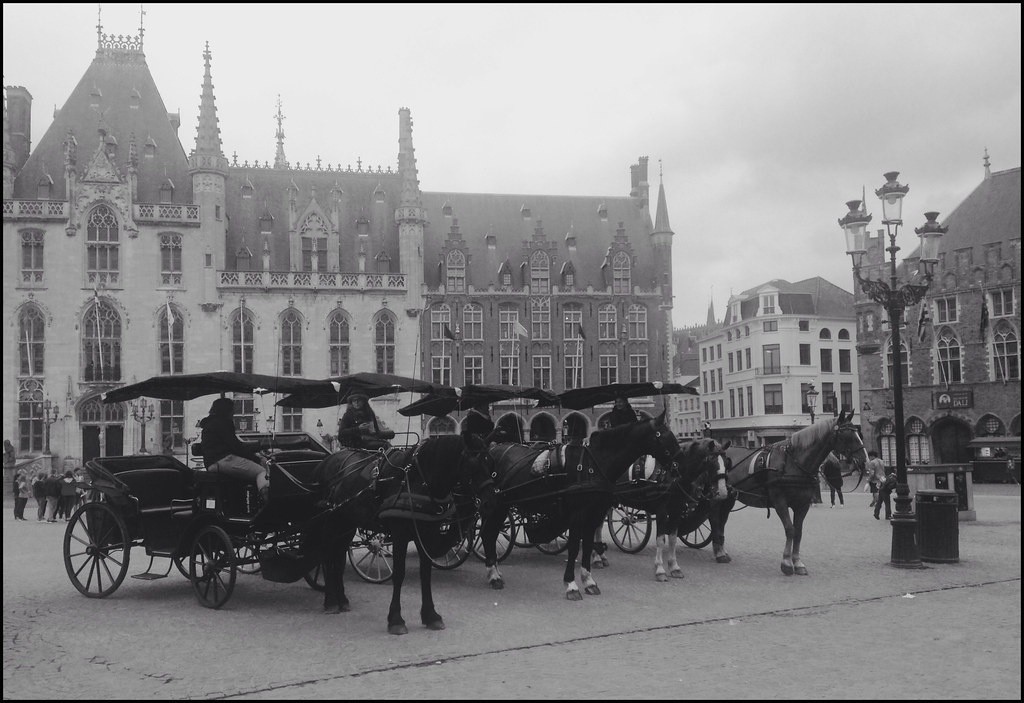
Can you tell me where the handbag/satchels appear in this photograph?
[864,483,870,492]
[880,475,887,482]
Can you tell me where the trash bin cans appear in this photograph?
[914,489,960,564]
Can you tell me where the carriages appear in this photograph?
[61,370,492,636]
[392,380,865,587]
[275,362,694,603]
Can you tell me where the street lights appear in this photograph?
[839,172,950,570]
[130,396,155,457]
[36,399,60,455]
[806,385,820,426]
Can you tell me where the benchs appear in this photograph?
[86,430,337,551]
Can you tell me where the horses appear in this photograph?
[312,408,868,637]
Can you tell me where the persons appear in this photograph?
[866,451,897,520]
[609,390,638,428]
[13,467,88,524]
[338,388,396,450]
[461,397,494,437]
[823,452,844,509]
[993,449,1006,458]
[202,398,270,501]
[3,439,16,465]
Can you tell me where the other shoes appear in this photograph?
[840,505,844,508]
[830,505,836,509]
[870,501,876,507]
[886,516,894,519]
[874,514,880,520]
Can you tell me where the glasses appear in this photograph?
[352,398,363,402]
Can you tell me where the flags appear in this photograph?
[578,325,586,339]
[514,321,529,338]
[444,324,453,339]
[979,302,990,341]
[917,299,930,343]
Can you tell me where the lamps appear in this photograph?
[36,400,58,455]
[131,397,155,453]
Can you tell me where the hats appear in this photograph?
[345,387,369,402]
[209,398,234,412]
